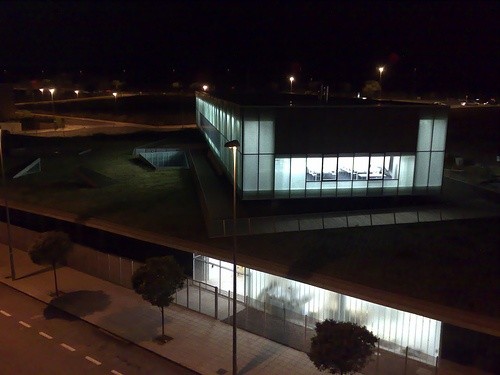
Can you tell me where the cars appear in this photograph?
[460,94,496,108]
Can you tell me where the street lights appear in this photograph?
[38,88,119,118]
[287,65,386,98]
[224,139,240,375]
